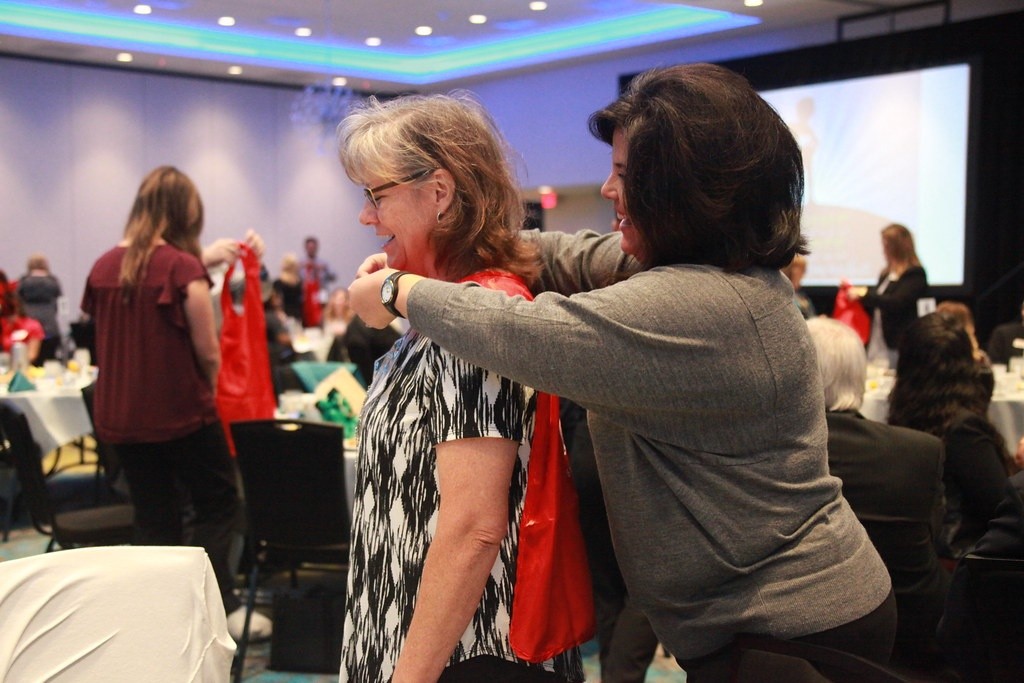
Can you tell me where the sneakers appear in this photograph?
[228,606,273,642]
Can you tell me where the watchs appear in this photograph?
[380,271,410,319]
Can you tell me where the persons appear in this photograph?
[886,302,1024,533]
[987,302,1024,364]
[847,224,928,369]
[938,469,1024,683]
[335,93,596,683]
[261,237,401,384]
[0,287,45,362]
[347,63,897,683]
[595,595,658,683]
[808,316,949,670]
[15,255,62,362]
[81,165,270,640]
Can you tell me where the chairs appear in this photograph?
[0,321,350,683]
[683,513,1024,683]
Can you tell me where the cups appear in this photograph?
[992,357,1024,391]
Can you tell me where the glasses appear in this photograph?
[365,169,424,209]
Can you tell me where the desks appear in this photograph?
[857,374,1024,457]
[0,365,98,473]
